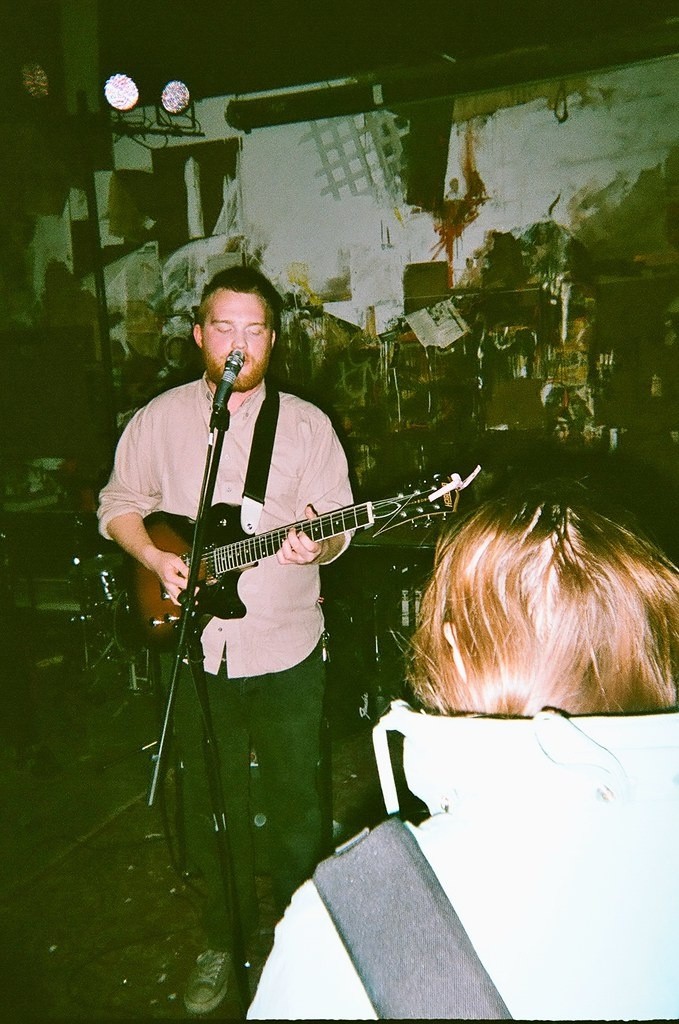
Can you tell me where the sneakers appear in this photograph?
[181,947,233,1016]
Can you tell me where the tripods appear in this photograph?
[58,590,108,671]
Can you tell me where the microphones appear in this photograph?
[211,350,245,416]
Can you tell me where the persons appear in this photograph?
[245,488,679,1019]
[97,267,358,1015]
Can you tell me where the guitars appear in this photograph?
[112,463,489,658]
